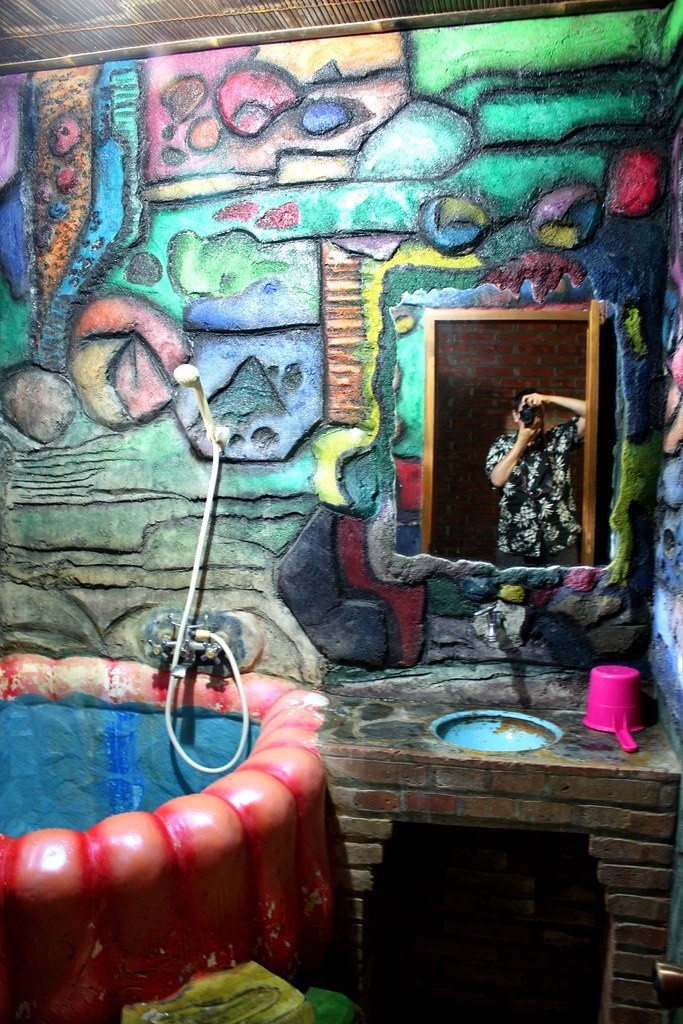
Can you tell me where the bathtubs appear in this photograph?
[0,648,346,1024]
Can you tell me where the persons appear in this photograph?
[485,388,586,569]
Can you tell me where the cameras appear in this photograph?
[518,403,540,428]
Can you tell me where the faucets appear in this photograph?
[170,638,196,681]
[472,604,503,643]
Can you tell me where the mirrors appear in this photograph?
[421,299,599,568]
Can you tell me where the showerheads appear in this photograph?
[173,363,219,443]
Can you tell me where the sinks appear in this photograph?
[429,706,565,753]
